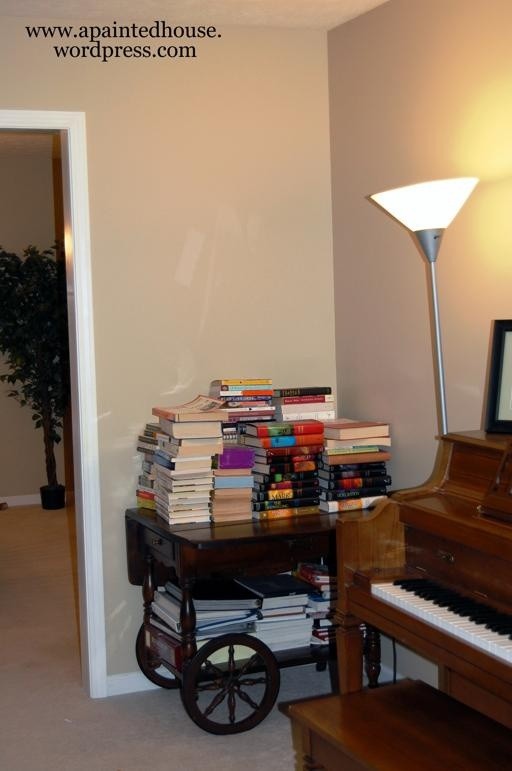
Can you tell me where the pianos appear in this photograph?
[334,434,511,729]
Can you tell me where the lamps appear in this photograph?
[370,177,480,434]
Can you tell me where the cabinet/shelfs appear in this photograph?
[124,507,381,735]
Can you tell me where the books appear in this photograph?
[150,562,367,668]
[136,379,392,526]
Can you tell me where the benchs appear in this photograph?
[279,679,512,770]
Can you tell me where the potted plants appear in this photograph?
[0,232,69,510]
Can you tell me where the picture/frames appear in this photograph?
[484,320,512,435]
